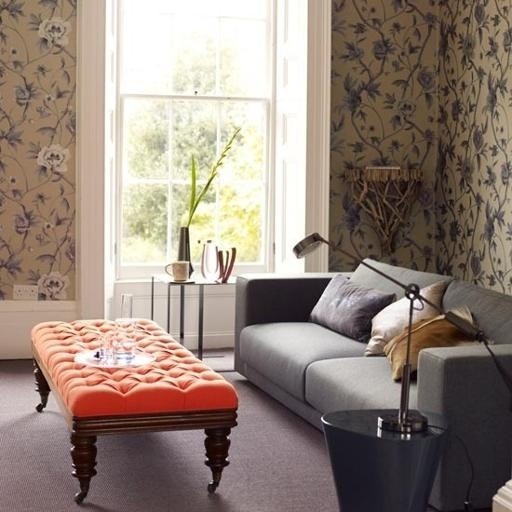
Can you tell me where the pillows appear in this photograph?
[383,304,495,380]
[310,273,395,344]
[365,280,450,357]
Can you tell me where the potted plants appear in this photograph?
[177,125,241,277]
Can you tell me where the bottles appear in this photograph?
[203,240,220,283]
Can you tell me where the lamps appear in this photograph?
[292,233,512,433]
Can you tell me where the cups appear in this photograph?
[164,261,190,281]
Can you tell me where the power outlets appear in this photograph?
[13,286,39,300]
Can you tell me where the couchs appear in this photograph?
[233,257,512,512]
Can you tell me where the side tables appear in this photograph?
[319,409,457,512]
[150,271,236,360]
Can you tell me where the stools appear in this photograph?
[31,317,239,503]
